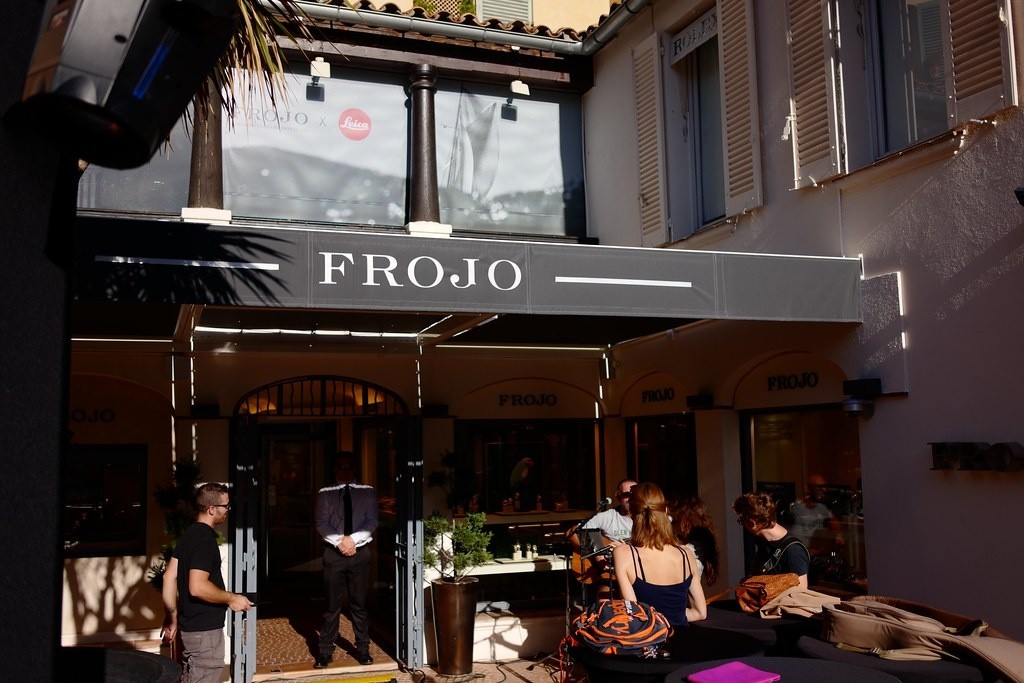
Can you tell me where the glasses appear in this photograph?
[205,503,231,510]
[738,517,749,525]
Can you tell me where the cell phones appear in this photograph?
[236,593,258,607]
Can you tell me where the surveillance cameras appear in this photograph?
[843,404,864,418]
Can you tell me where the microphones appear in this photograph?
[598,497,612,505]
[668,516,672,522]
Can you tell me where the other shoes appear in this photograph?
[358,649,373,665]
[314,654,334,668]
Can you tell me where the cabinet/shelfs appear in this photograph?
[451,507,594,617]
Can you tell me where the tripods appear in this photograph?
[527,504,607,672]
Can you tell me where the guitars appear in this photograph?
[571,531,632,584]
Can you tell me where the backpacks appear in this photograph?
[558,598,673,681]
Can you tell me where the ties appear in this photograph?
[344,485,353,537]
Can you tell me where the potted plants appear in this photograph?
[419,509,495,676]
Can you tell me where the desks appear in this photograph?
[666,599,991,683]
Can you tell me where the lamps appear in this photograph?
[510,80,530,96]
[310,57,330,78]
[191,404,221,418]
[421,402,449,417]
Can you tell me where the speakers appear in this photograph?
[21,0,240,169]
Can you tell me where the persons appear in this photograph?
[314,451,378,669]
[422,465,455,516]
[566,479,675,574]
[670,492,717,583]
[613,482,707,640]
[790,474,848,550]
[732,492,810,591]
[163,483,254,683]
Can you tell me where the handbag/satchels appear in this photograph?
[734,573,798,613]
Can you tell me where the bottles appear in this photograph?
[533,545,538,559]
[537,496,542,511]
[502,499,513,513]
[527,546,532,559]
[513,545,522,560]
[458,506,464,515]
